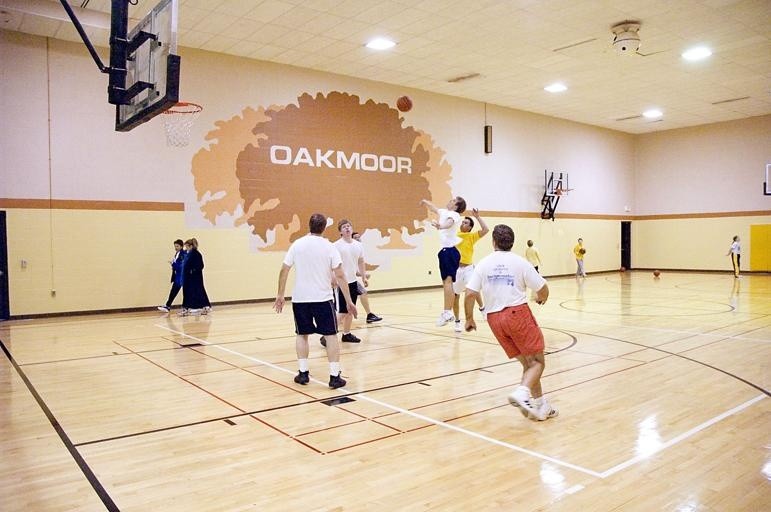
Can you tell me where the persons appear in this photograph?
[176,239,212,316]
[157,239,202,313]
[725,236,743,279]
[272,213,358,390]
[320,219,369,347]
[464,224,559,421]
[420,196,466,327]
[525,240,543,273]
[352,233,382,323]
[452,208,489,332]
[574,239,588,277]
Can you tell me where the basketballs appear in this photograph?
[580,248,585,254]
[620,266,626,272]
[396,95,412,112]
[654,270,660,276]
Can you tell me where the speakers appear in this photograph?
[485,125,492,153]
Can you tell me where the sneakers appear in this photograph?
[200,306,212,314]
[435,312,454,326]
[534,403,559,421]
[329,371,346,387]
[158,305,170,313]
[320,336,326,346]
[190,309,201,313]
[176,308,188,317]
[294,369,309,384]
[366,313,382,323]
[342,333,360,343]
[507,389,540,421]
[455,322,462,332]
[480,306,488,319]
